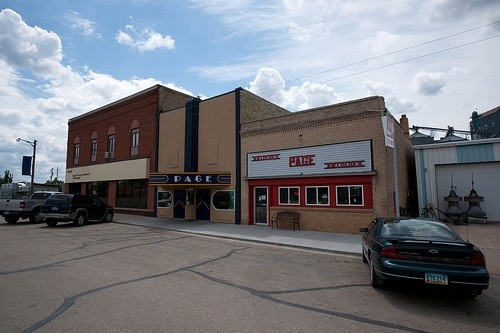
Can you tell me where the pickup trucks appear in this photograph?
[0,191,73,224]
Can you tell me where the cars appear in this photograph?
[359,217,489,302]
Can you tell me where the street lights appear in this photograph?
[16,138,37,195]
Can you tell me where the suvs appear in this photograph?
[37,192,114,227]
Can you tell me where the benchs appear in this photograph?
[271,211,300,232]
